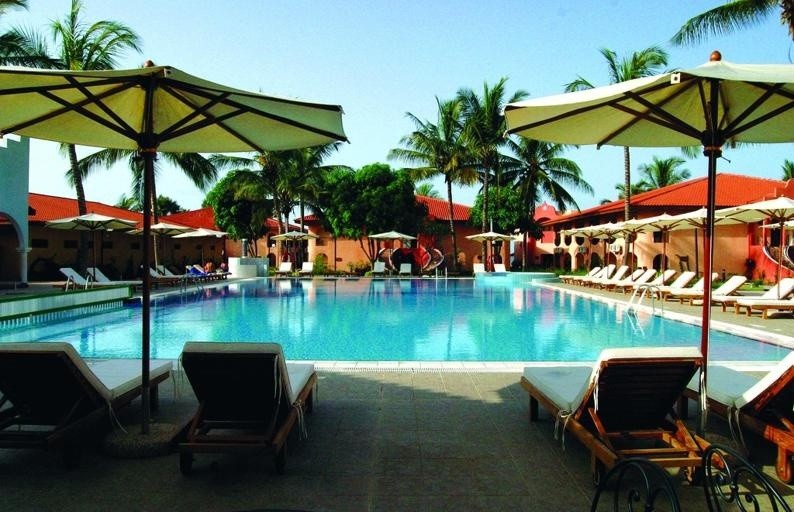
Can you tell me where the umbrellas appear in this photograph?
[270,231,319,268]
[557,194,794,299]
[171,226,229,268]
[368,230,417,264]
[0,60,349,436]
[126,222,193,273]
[44,210,140,279]
[503,53,794,439]
[465,232,516,272]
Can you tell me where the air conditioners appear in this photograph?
[610,244,620,252]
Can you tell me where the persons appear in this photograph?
[282,243,300,264]
[204,247,228,273]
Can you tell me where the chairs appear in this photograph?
[676,350,794,483]
[0,341,173,468]
[399,263,412,277]
[170,342,318,476]
[493,263,511,276]
[519,346,730,485]
[559,264,794,319]
[371,262,386,278]
[59,264,232,292]
[299,261,314,277]
[275,262,293,278]
[472,263,487,277]
